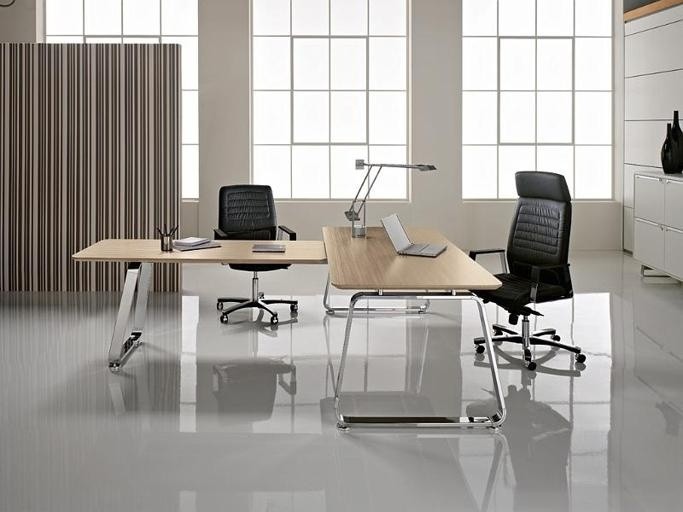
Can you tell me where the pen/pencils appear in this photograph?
[157,224,179,236]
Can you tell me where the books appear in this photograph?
[173,236,221,251]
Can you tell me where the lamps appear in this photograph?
[345,159,438,239]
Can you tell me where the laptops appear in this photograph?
[380,213,447,258]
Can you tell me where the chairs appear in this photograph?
[214,185,298,329]
[470,172,587,367]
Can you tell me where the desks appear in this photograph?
[71,237,327,372]
[323,225,508,429]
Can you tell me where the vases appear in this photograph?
[661,110,683,175]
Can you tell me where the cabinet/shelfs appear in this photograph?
[623,0,683,252]
[632,172,683,282]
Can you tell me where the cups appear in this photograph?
[161,234,172,251]
